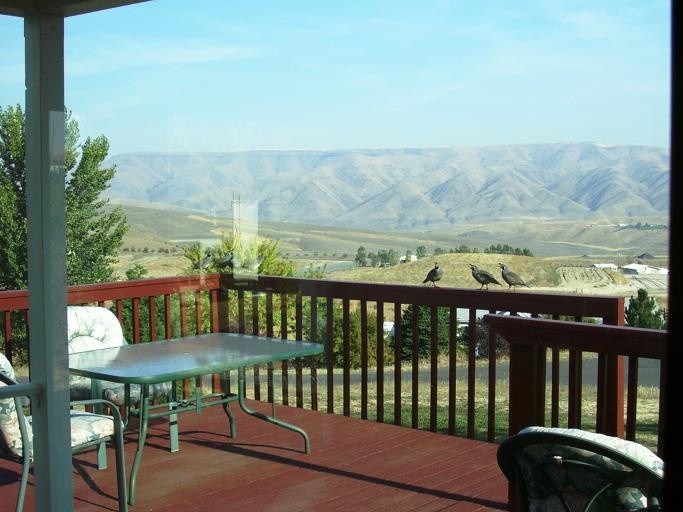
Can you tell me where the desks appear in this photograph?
[67,332,325,507]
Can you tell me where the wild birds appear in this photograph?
[498,262,527,292]
[423,262,444,288]
[469,264,503,291]
[188,250,267,275]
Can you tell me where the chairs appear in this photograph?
[495,425,664,510]
[67,305,180,469]
[0,351,128,511]
